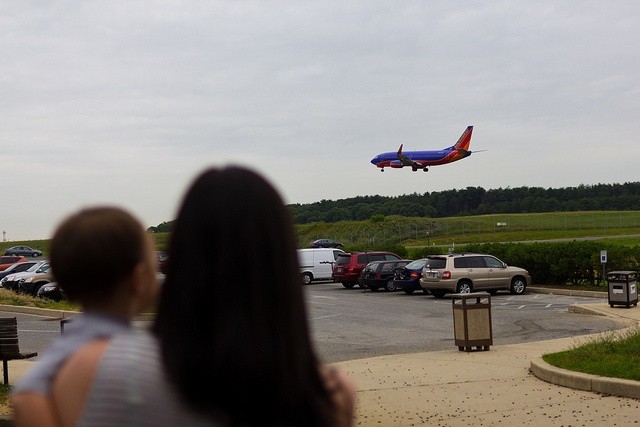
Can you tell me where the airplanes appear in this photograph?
[371,126,488,172]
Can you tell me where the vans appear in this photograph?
[296,248,347,285]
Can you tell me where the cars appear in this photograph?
[149,250,169,274]
[19,265,56,296]
[0,262,44,288]
[393,258,434,295]
[310,239,343,249]
[0,256,28,271]
[3,260,51,291]
[37,281,68,302]
[5,245,43,258]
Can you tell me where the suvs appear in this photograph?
[420,252,532,298]
[333,251,407,289]
[358,260,413,291]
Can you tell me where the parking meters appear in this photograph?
[601,249,607,287]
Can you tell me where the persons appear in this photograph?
[51,162,356,427]
[12,205,157,427]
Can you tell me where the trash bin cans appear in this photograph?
[451,293,493,351]
[607,271,638,308]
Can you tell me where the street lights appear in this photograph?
[426,232,429,249]
